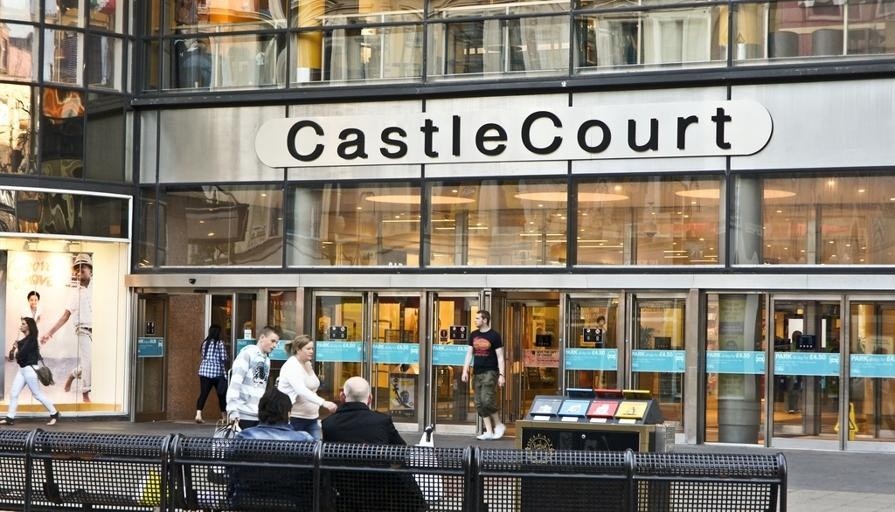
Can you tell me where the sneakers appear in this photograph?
[477,432,494,440]
[9,350,14,359]
[493,423,507,440]
[195,415,206,424]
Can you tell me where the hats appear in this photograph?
[73,253,92,270]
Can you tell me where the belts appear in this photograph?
[80,327,92,332]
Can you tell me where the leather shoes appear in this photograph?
[47,412,62,425]
[0,416,15,425]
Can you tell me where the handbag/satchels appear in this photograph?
[37,366,54,386]
[213,420,242,439]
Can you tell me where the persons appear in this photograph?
[320,376,431,511]
[194,323,227,424]
[460,306,507,443]
[786,330,805,416]
[8,290,42,361]
[593,314,607,388]
[41,252,95,404]
[224,325,280,433]
[0,316,60,427]
[275,334,340,443]
[222,387,323,511]
